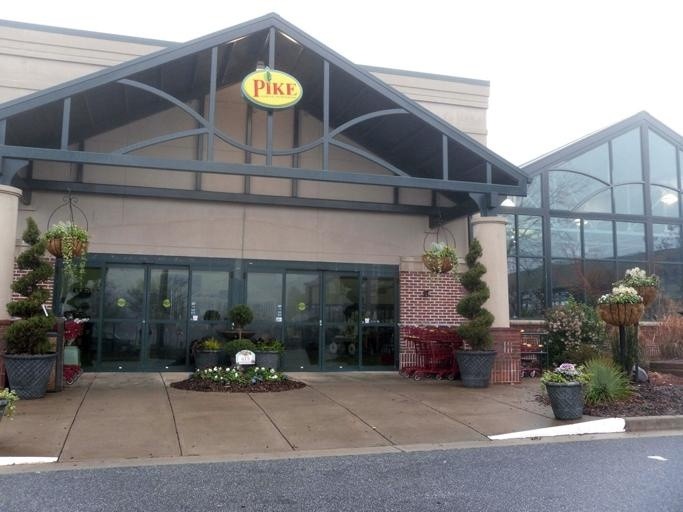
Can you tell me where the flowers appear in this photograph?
[540,361,592,388]
[597,267,659,307]
[423,242,459,282]
[45,222,89,290]
[0,387,19,422]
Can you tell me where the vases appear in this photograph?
[598,303,646,327]
[423,254,454,274]
[47,238,86,259]
[545,381,588,420]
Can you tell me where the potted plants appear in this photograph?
[0,216,66,399]
[451,238,498,388]
[192,299,285,374]
